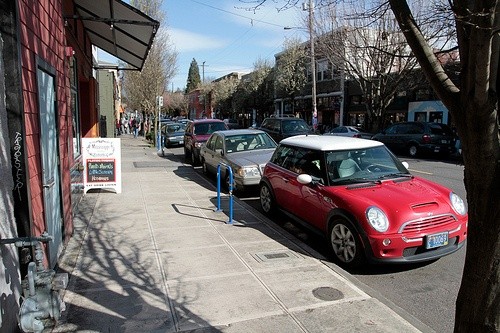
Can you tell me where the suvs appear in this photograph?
[260,117,316,144]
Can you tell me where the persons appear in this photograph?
[295,111,300,118]
[116,111,142,138]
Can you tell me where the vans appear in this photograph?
[374,121,460,158]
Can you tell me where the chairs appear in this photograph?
[336,158,361,178]
[247,138,258,149]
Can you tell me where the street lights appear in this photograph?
[283,26,319,133]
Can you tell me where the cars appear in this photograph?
[152,117,190,127]
[323,127,372,140]
[259,134,469,269]
[160,122,186,148]
[183,118,231,165]
[200,128,281,196]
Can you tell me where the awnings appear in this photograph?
[64,0,161,72]
